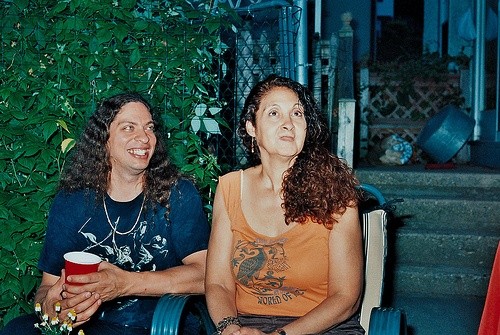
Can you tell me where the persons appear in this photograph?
[205,74,366,335]
[3,92,208,335]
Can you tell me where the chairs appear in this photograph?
[150,183,401,335]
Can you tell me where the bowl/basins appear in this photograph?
[417,105,476,164]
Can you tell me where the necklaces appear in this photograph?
[101,191,147,236]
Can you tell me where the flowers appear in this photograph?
[34,302,85,335]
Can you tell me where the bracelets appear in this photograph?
[274,327,287,335]
[216,316,241,335]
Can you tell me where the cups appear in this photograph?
[64,252,101,289]
